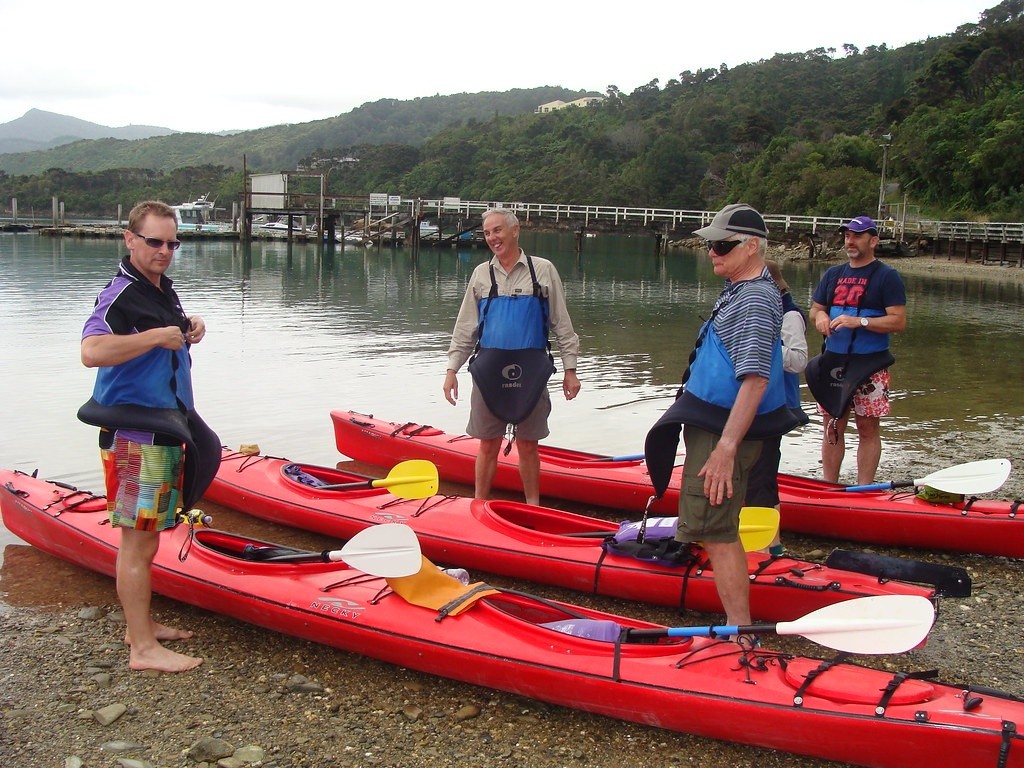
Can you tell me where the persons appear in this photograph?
[744,259,808,559]
[809,217,907,488]
[443,207,581,506]
[674,203,782,646]
[80,201,203,674]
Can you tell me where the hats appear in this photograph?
[692,203,769,242]
[837,216,879,238]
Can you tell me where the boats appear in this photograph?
[0,467,1024,768]
[259,223,310,233]
[203,444,975,622]
[168,191,239,237]
[875,194,924,257]
[331,410,1024,559]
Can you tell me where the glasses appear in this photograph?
[134,233,181,251]
[705,240,742,257]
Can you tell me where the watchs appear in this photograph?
[860,316,868,329]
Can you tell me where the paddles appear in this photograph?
[585,453,646,459]
[258,522,422,578]
[825,459,1011,496]
[531,592,934,657]
[555,506,779,553]
[314,457,438,499]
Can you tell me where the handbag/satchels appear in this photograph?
[597,517,692,566]
